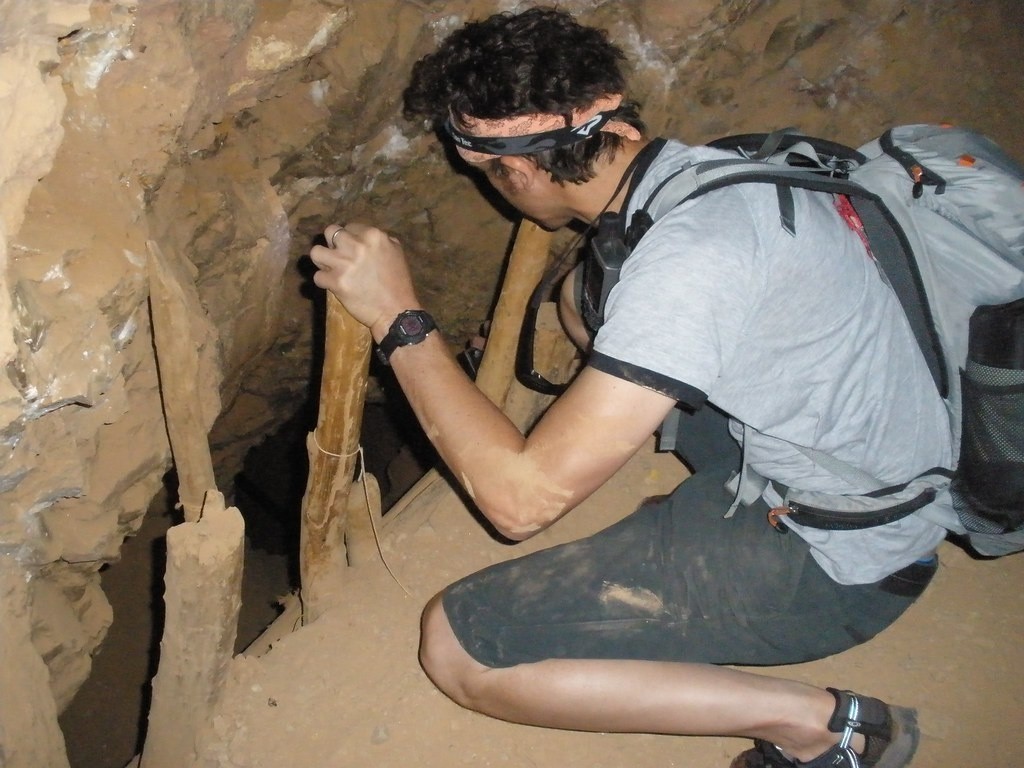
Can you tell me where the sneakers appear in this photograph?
[728,685,920,768]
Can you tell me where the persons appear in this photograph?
[311,6,953,768]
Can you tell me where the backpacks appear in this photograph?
[843,120,1024,562]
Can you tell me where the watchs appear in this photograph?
[376,310,440,364]
